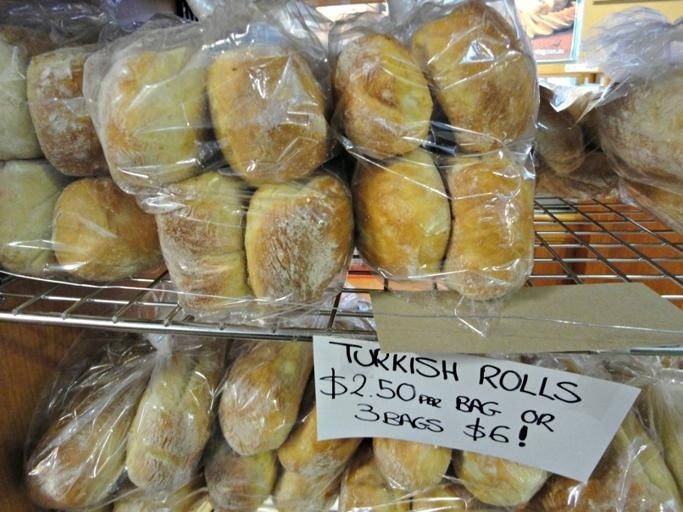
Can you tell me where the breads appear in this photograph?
[21,339,683,512]
[1,2,683,316]
